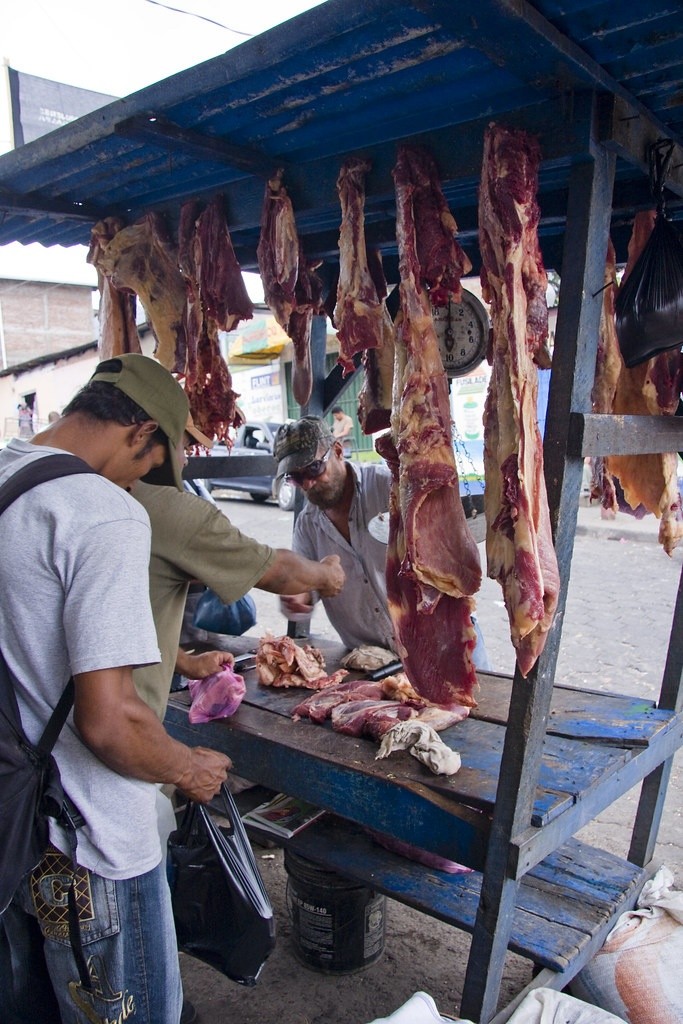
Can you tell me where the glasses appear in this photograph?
[285,448,332,487]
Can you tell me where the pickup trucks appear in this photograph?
[193,422,296,510]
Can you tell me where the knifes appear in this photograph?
[234,648,258,662]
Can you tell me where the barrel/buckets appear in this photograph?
[283,846,387,976]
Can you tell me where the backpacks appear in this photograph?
[0,454,104,914]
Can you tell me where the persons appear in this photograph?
[0,357,342,1024]
[273,412,492,673]
[330,406,354,454]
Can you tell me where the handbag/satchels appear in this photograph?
[194,580,256,636]
[163,780,276,986]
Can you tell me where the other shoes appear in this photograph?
[180,998,196,1024]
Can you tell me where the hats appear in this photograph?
[181,407,214,450]
[91,353,185,493]
[272,415,334,479]
